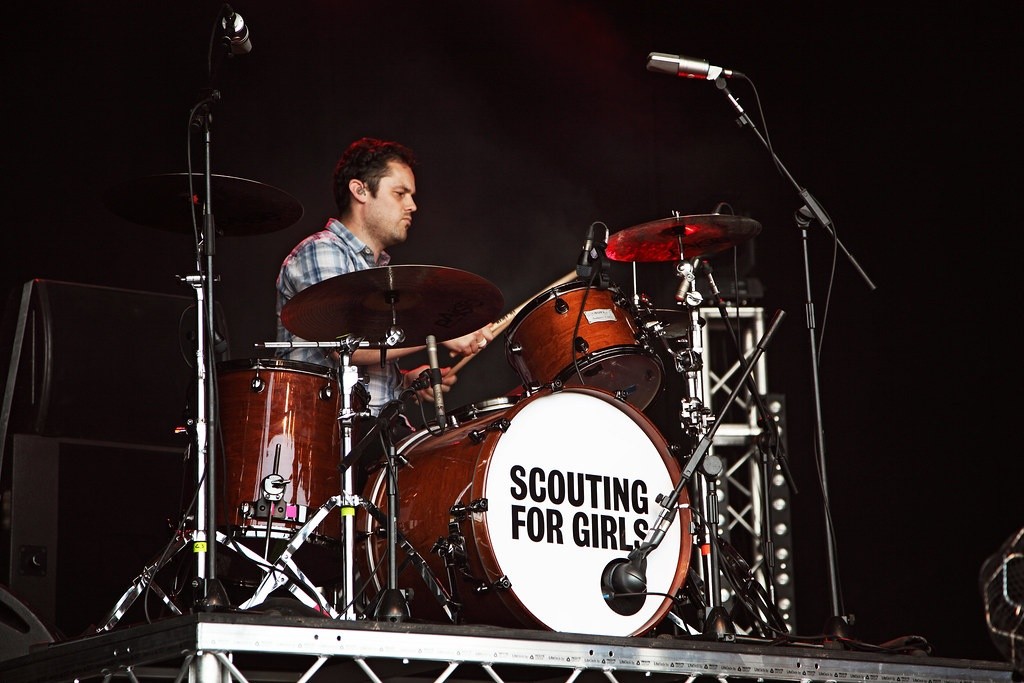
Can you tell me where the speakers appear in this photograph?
[0,280,236,639]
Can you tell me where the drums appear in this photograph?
[210,358,371,551]
[506,281,667,416]
[354,384,693,638]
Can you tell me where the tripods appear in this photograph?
[94,13,467,637]
[665,76,928,658]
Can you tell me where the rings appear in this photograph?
[478,338,487,348]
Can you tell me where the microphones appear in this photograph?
[646,52,745,80]
[577,223,597,267]
[222,4,253,55]
[676,203,724,302]
[425,335,446,431]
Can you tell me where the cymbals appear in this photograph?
[280,265,504,350]
[602,214,763,263]
[103,173,304,240]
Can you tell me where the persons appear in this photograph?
[276,138,494,496]
